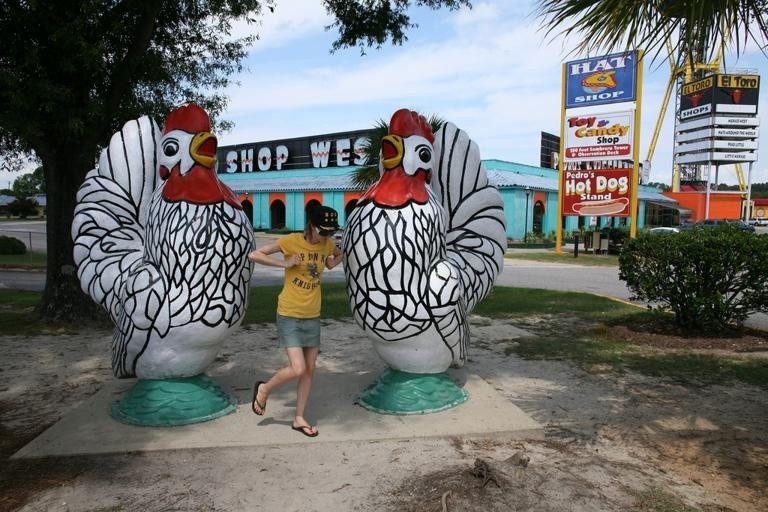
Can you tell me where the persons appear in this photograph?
[248,206,345,437]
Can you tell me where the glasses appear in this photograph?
[319,229,339,236]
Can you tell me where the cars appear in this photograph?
[647,215,768,236]
[330,227,344,251]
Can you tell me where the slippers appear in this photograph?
[252,381,266,415]
[292,422,319,437]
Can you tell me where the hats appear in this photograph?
[310,206,340,230]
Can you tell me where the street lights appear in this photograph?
[522,185,531,245]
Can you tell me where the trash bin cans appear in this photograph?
[584,231,600,254]
